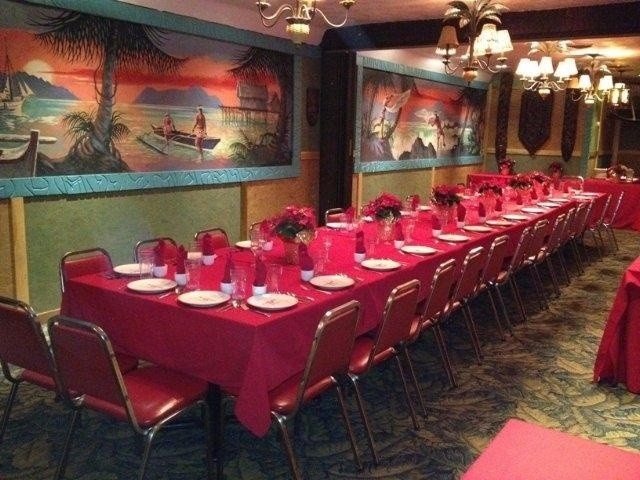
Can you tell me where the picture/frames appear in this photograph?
[0,0,307,198]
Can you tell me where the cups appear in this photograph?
[154,263,169,278]
[220,278,236,294]
[252,283,268,297]
[228,262,249,309]
[136,246,157,279]
[184,260,204,293]
[174,271,193,287]
[262,240,274,251]
[301,269,314,281]
[250,229,264,268]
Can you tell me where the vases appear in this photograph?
[354,57,484,175]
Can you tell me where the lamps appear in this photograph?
[436,1,614,105]
[256,0,355,45]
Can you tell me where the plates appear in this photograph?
[307,185,599,293]
[126,276,176,298]
[176,288,232,311]
[114,263,156,276]
[235,240,257,250]
[245,291,300,314]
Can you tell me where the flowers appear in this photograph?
[477,177,503,198]
[514,169,549,188]
